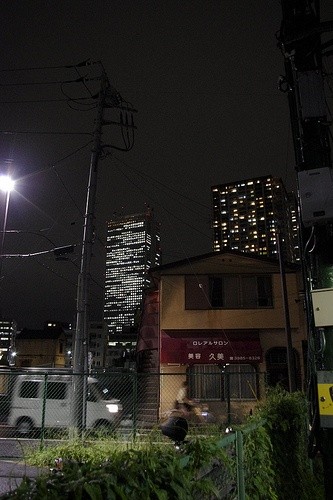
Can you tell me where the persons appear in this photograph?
[173,381,200,416]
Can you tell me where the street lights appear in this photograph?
[0,174,15,255]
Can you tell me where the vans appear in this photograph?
[9,372,124,441]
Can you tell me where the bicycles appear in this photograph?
[171,398,209,425]
